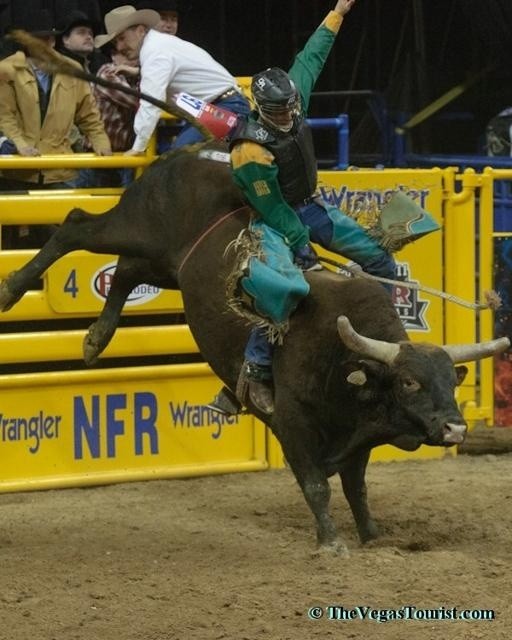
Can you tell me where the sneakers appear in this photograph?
[247,362,274,414]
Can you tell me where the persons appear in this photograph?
[226,1,398,419]
[483,288,512,337]
[0,1,254,333]
[484,85,512,160]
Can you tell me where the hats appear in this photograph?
[52,10,95,36]
[94,5,160,49]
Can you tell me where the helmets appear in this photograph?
[251,67,301,115]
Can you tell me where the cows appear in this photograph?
[0,29,511,550]
